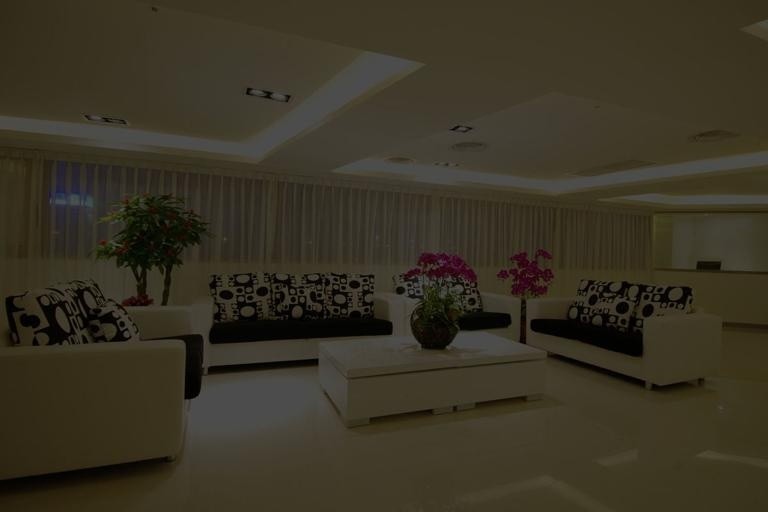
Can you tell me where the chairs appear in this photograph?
[389,291,522,341]
[0,305,194,482]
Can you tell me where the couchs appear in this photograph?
[192,290,408,377]
[524,297,722,391]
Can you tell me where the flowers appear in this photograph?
[87,193,216,305]
[403,249,479,314]
[497,249,554,298]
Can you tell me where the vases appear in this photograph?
[409,309,460,350]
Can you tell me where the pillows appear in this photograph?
[4,275,143,348]
[206,271,376,325]
[565,278,694,334]
